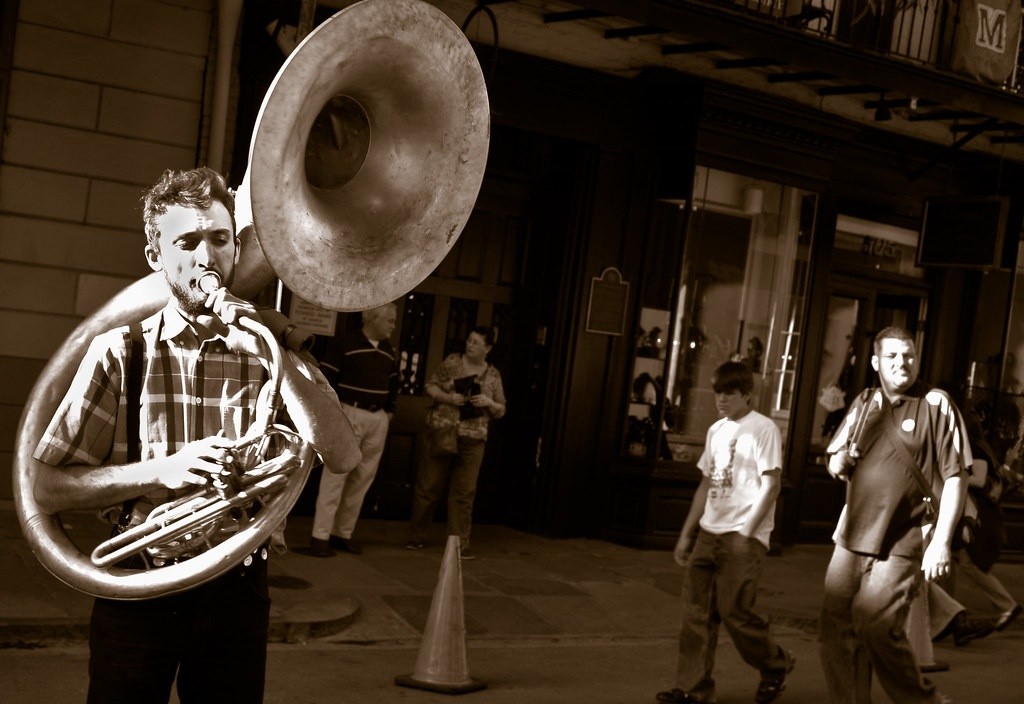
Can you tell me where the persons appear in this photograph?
[819,326,974,704]
[406,324,506,559]
[32,163,362,704]
[655,359,796,704]
[310,301,400,557]
[934,390,1022,630]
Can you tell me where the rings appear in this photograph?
[456,400,460,403]
[478,400,482,403]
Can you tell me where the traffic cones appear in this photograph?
[394,535,486,695]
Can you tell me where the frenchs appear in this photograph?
[11,1,492,602]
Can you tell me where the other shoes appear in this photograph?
[406,540,423,547]
[330,536,360,553]
[756,654,797,704]
[657,689,718,704]
[310,539,336,555]
[919,660,949,671]
[997,602,1024,628]
[954,616,999,644]
[462,550,476,559]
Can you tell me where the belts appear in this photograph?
[338,398,385,413]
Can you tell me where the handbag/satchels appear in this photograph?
[429,426,458,457]
[454,374,485,422]
